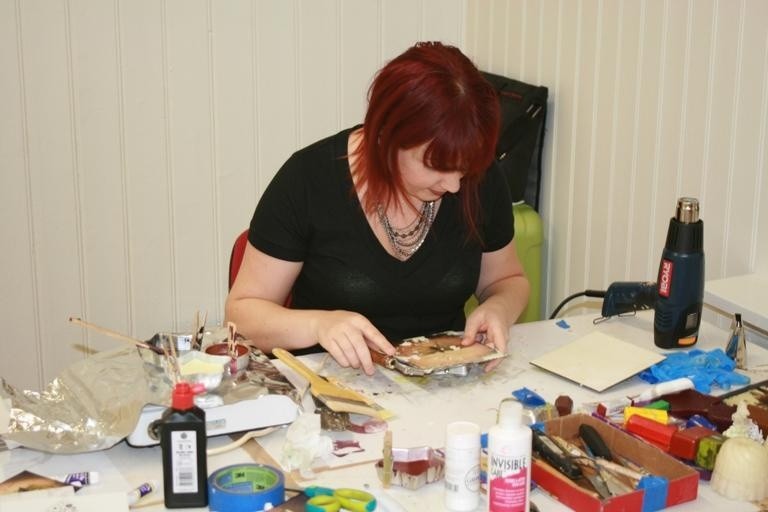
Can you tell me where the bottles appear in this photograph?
[158,381,209,509]
[486,398,533,512]
[441,421,482,512]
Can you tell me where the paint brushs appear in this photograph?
[327,376,395,420]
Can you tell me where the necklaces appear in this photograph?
[374,193,438,260]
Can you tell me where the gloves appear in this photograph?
[639,350,750,393]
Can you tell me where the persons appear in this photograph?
[223,41,532,378]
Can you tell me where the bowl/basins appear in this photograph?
[202,341,250,383]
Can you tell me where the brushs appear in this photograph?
[271,347,378,418]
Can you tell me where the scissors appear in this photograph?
[284,481,377,512]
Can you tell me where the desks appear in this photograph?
[2,315,768,510]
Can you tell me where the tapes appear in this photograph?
[206,463,285,512]
